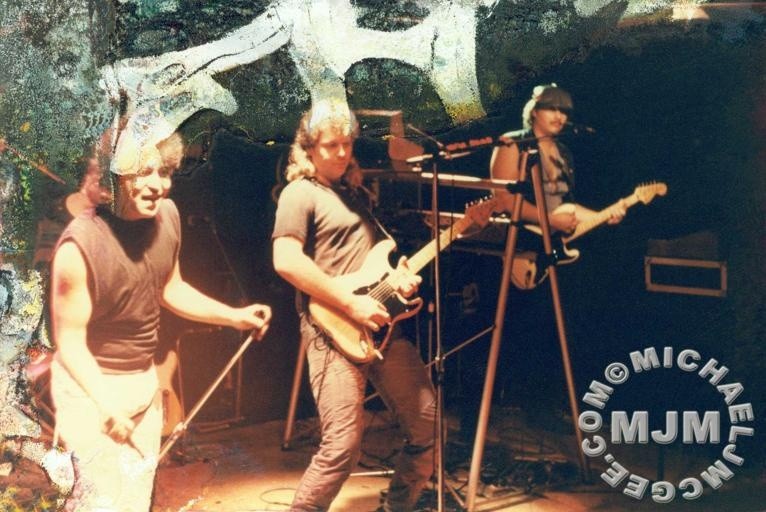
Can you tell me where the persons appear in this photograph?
[491,85,626,235]
[271,100,448,512]
[51,116,272,512]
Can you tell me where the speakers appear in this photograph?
[630,292,766,471]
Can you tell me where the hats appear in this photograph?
[532,82,574,111]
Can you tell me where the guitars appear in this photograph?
[306,196,498,363]
[153,349,182,438]
[502,181,670,292]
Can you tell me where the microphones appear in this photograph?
[406,125,444,150]
[565,122,595,133]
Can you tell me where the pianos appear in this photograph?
[394,208,511,244]
